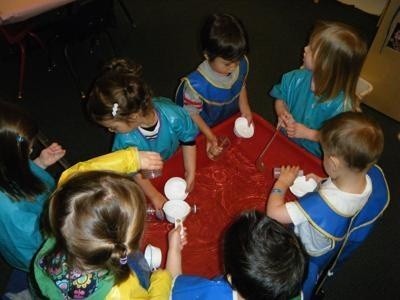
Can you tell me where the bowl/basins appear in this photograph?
[233,117,254,138]
[289,175,317,199]
[163,176,189,202]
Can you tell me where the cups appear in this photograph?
[206,134,230,162]
[142,171,162,179]
[273,167,304,179]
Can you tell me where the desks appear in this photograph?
[130,108,336,284]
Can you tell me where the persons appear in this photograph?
[264,110,392,268]
[268,12,368,160]
[169,208,310,300]
[81,56,199,221]
[174,10,256,160]
[0,99,68,274]
[28,146,189,300]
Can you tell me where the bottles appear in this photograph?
[145,202,200,221]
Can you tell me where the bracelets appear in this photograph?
[270,188,285,193]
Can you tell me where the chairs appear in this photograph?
[0,0,138,101]
